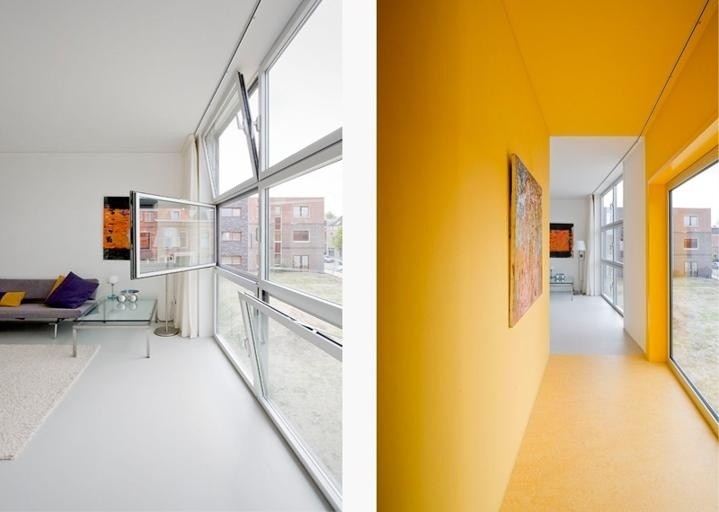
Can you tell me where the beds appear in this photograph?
[72,294,159,359]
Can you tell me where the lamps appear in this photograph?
[106,275,119,300]
[573,239,588,295]
[152,225,181,337]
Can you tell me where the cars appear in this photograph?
[160,244,189,265]
[324,254,335,263]
[269,263,290,273]
[712,259,719,269]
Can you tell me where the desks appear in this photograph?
[550,277,574,301]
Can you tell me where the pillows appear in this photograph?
[0,291,25,308]
[47,275,64,298]
[46,272,100,310]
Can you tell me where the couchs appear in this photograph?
[1,279,101,338]
